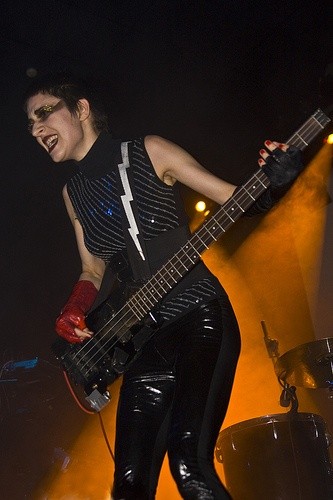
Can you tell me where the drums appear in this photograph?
[274,337,332,389]
[215,412,333,500]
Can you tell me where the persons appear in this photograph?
[22,75,300,500]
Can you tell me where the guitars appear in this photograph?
[51,109,330,398]
